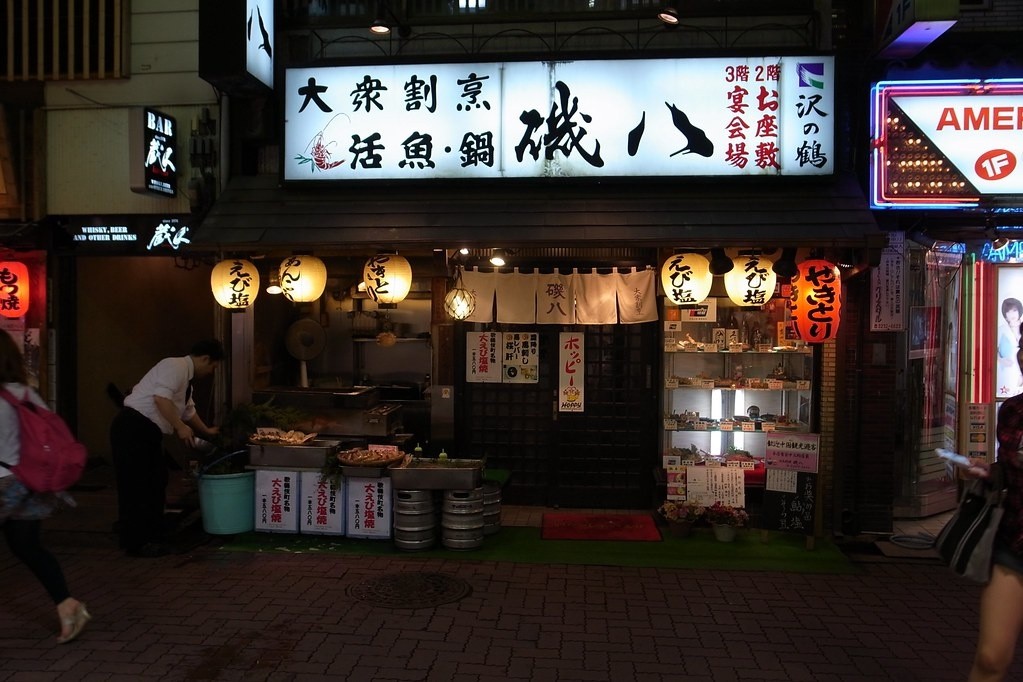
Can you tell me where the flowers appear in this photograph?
[659,499,707,524]
[705,500,750,528]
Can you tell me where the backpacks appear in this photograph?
[0,387,88,494]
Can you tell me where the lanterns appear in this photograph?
[790,259,841,346]
[722,255,778,310]
[278,257,328,304]
[364,254,413,310]
[1,262,31,318]
[212,259,258,313]
[661,254,714,310]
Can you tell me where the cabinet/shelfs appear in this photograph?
[350,279,433,388]
[658,295,818,488]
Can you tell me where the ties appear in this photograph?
[185,380,192,406]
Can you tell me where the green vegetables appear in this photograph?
[226,393,306,432]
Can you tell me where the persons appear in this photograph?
[967,329,1023,682]
[0,330,92,645]
[108,339,225,552]
[999,299,1023,353]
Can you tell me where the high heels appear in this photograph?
[57,605,91,644]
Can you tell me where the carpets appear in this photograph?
[217,525,868,577]
[540,512,664,542]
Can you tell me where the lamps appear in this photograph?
[709,247,736,274]
[914,227,936,249]
[443,245,475,320]
[984,219,1008,251]
[489,248,516,266]
[836,246,853,269]
[658,4,682,29]
[264,267,282,295]
[370,2,413,39]
[772,247,798,277]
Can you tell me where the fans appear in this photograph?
[285,317,327,387]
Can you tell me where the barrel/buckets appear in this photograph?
[191,450,256,535]
[389,480,503,551]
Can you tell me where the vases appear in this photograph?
[667,519,691,538]
[712,524,738,543]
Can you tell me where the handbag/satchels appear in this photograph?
[933,463,1008,584]
